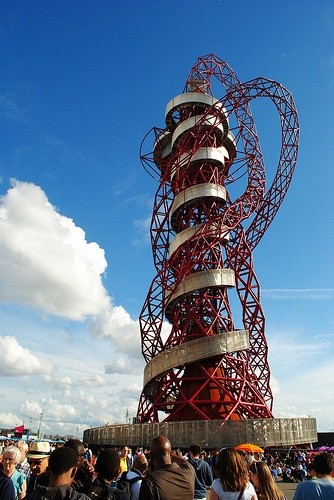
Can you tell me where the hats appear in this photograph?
[25,442,51,459]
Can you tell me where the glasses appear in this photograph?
[77,451,86,458]
[253,461,259,475]
[26,457,46,465]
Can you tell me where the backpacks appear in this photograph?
[115,471,143,500]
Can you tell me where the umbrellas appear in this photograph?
[235,443,264,453]
[315,445,334,452]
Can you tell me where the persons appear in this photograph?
[62,439,94,495]
[3,447,27,500]
[76,448,129,500]
[209,448,257,500]
[119,445,128,474]
[0,445,15,500]
[21,448,91,500]
[26,440,33,450]
[134,435,196,500]
[186,444,220,500]
[292,453,334,500]
[250,461,288,500]
[0,439,19,446]
[237,445,315,483]
[50,441,63,452]
[25,442,51,495]
[117,455,147,500]
[15,440,30,475]
